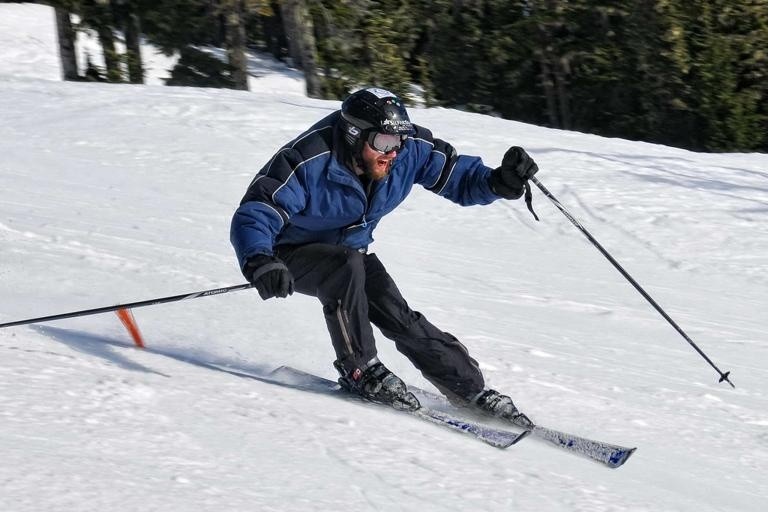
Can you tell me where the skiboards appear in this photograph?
[267,364,637,469]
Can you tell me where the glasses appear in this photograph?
[368,130,408,154]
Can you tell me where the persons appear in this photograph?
[229,88,541,425]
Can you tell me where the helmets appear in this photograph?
[342,88,416,151]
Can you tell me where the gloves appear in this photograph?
[243,255,294,300]
[492,146,538,200]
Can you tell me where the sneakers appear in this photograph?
[334,356,406,402]
[448,389,514,417]
